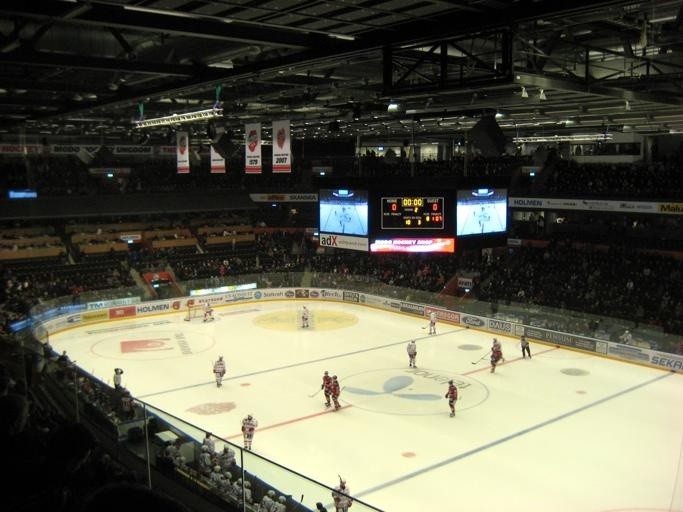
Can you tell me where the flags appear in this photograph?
[175,130,191,173]
[245,123,262,175]
[272,119,292,172]
[211,144,226,174]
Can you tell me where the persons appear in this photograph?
[320,370,332,407]
[519,335,531,359]
[1,215,682,340]
[329,375,341,412]
[316,502,327,512]
[489,336,502,372]
[406,339,417,368]
[0,340,304,510]
[444,379,457,417]
[331,478,351,512]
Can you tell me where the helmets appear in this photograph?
[448,379,453,384]
[340,479,347,485]
[201,444,287,504]
[219,354,223,359]
[411,338,416,342]
[324,371,329,374]
[332,375,338,379]
[247,412,253,418]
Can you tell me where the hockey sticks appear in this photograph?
[336,475,343,510]
[472,350,492,364]
[308,389,322,397]
[422,324,430,329]
[290,495,304,511]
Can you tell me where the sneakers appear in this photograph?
[203,316,214,322]
[409,364,417,369]
[429,331,436,335]
[332,404,342,411]
[490,368,495,374]
[217,383,222,388]
[325,402,332,407]
[302,324,310,328]
[521,355,532,360]
[450,412,456,417]
